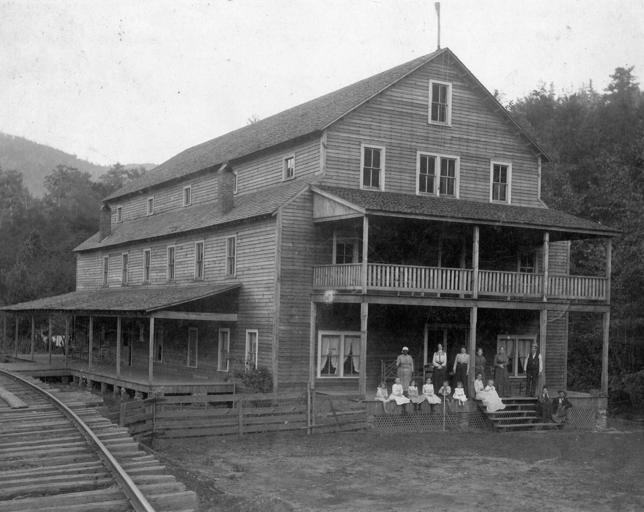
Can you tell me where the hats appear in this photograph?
[558,390,567,396]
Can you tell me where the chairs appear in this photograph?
[379,358,435,389]
[70,344,89,360]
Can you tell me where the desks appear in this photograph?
[501,375,528,395]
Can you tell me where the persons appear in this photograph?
[452,381,468,406]
[375,382,390,413]
[472,344,509,412]
[430,343,448,398]
[394,345,414,397]
[448,345,472,397]
[534,386,553,422]
[552,390,573,420]
[523,342,542,396]
[390,378,410,416]
[438,380,454,411]
[407,379,425,410]
[422,377,442,412]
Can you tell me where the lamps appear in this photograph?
[541,293,548,303]
